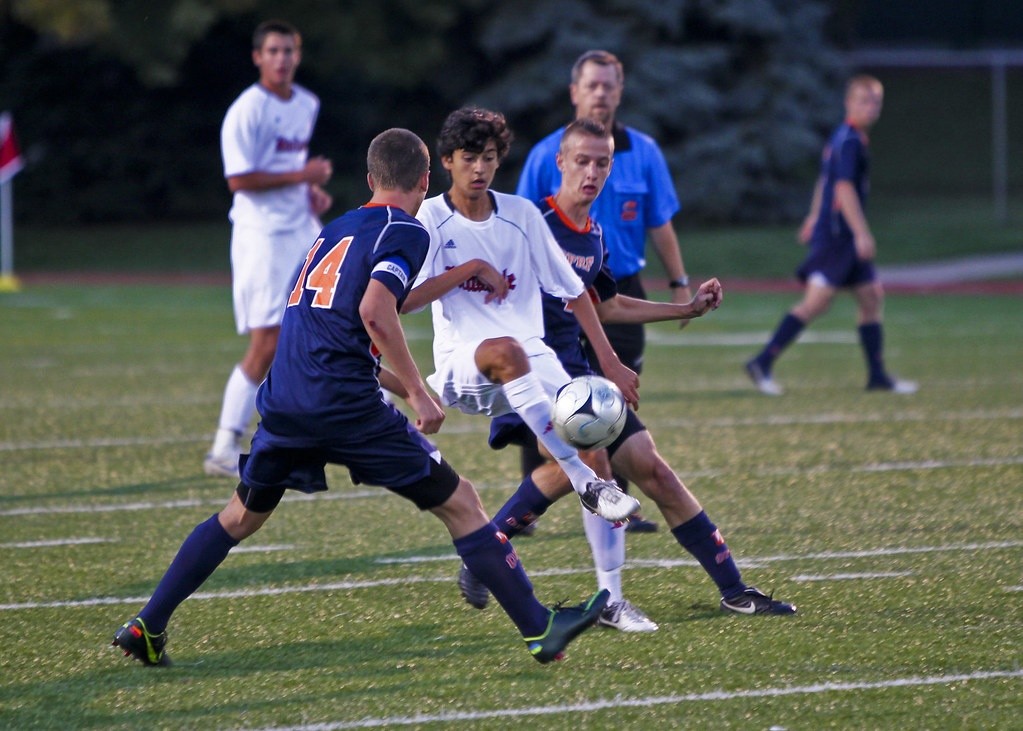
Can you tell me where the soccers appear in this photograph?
[551,374,629,454]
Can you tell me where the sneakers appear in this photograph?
[111,618,172,666]
[523,589,610,664]
[720,587,797,615]
[458,563,488,609]
[597,599,658,632]
[205,451,241,478]
[578,477,640,521]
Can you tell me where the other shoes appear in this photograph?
[625,514,658,532]
[865,381,920,394]
[525,521,539,531]
[746,360,785,396]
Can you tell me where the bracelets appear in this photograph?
[669,275,689,288]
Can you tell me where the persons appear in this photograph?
[745,74,919,397]
[202,23,392,478]
[459,119,798,615]
[114,129,610,665]
[515,49,693,537]
[399,105,658,634]
